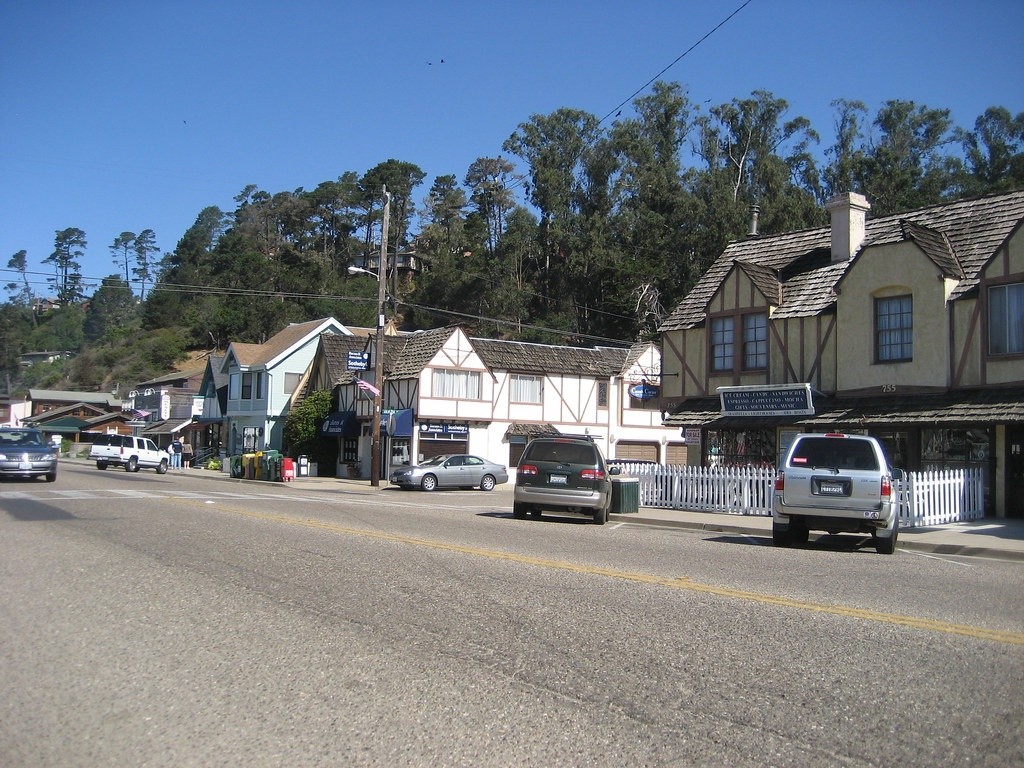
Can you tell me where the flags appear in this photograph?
[352,376,381,397]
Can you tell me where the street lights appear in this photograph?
[347,266,386,485]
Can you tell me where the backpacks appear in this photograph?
[168,444,175,455]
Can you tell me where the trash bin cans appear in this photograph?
[280,458,294,482]
[611,477,639,513]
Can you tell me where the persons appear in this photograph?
[182,438,194,470]
[172,437,183,470]
[168,441,175,467]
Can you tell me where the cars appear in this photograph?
[391,453,508,492]
[0,428,58,482]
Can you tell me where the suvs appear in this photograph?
[773,433,904,552]
[87,434,170,474]
[513,434,621,525]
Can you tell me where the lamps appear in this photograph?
[1011,444,1020,456]
